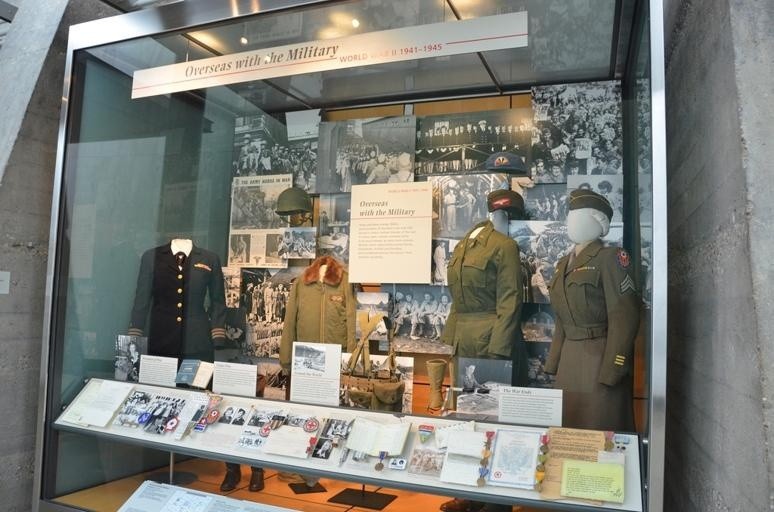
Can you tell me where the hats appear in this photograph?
[486,189,525,219]
[485,152,528,174]
[569,188,614,221]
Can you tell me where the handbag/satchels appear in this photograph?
[349,388,370,406]
[370,381,407,412]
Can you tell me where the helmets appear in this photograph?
[275,187,312,227]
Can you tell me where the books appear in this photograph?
[175,359,202,386]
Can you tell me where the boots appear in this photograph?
[426,358,446,411]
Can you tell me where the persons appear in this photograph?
[332,135,413,193]
[414,116,527,175]
[124,340,140,383]
[423,179,652,286]
[318,209,332,239]
[324,419,335,439]
[542,187,640,433]
[219,405,234,425]
[458,361,485,389]
[125,236,228,392]
[443,219,533,387]
[277,256,357,387]
[388,290,454,344]
[314,438,334,460]
[231,194,315,362]
[231,408,246,425]
[530,83,625,185]
[334,423,345,435]
[234,132,318,193]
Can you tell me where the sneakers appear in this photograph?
[249,468,266,491]
[220,470,241,491]
